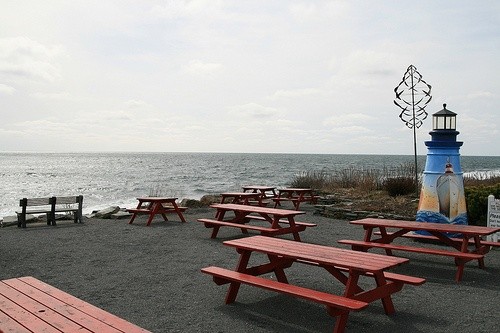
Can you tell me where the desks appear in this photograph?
[273,187,316,211]
[242,185,281,206]
[0,276,154,333]
[349,217,500,283]
[128,197,186,226]
[214,192,262,218]
[223,234,410,333]
[208,203,307,241]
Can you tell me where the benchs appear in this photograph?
[291,194,321,198]
[337,238,485,282]
[197,218,279,233]
[276,254,425,287]
[126,208,176,212]
[244,214,318,227]
[201,266,369,333]
[15,194,83,229]
[272,197,308,201]
[373,230,500,250]
[163,206,189,210]
